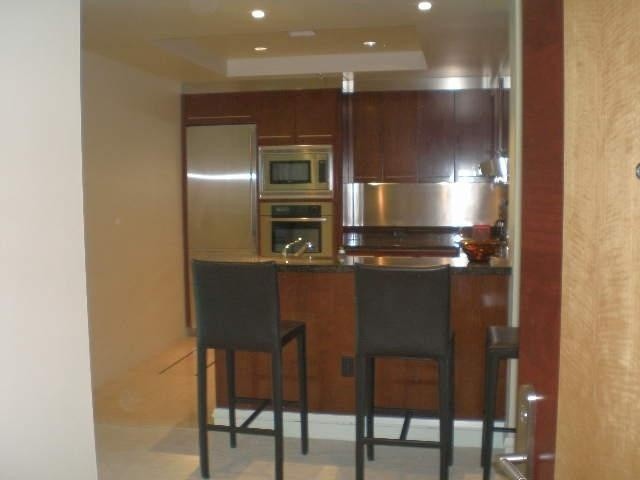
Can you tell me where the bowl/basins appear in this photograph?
[459,239,501,262]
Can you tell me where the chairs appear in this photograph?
[480,325,521,477]
[192,256,308,480]
[355,265,454,480]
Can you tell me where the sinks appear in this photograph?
[292,254,334,260]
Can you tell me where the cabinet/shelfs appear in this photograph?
[256,88,342,145]
[347,89,494,184]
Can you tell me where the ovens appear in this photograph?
[259,142,334,201]
[258,202,335,264]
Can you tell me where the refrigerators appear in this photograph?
[186,123,258,334]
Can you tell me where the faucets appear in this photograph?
[295,240,314,256]
